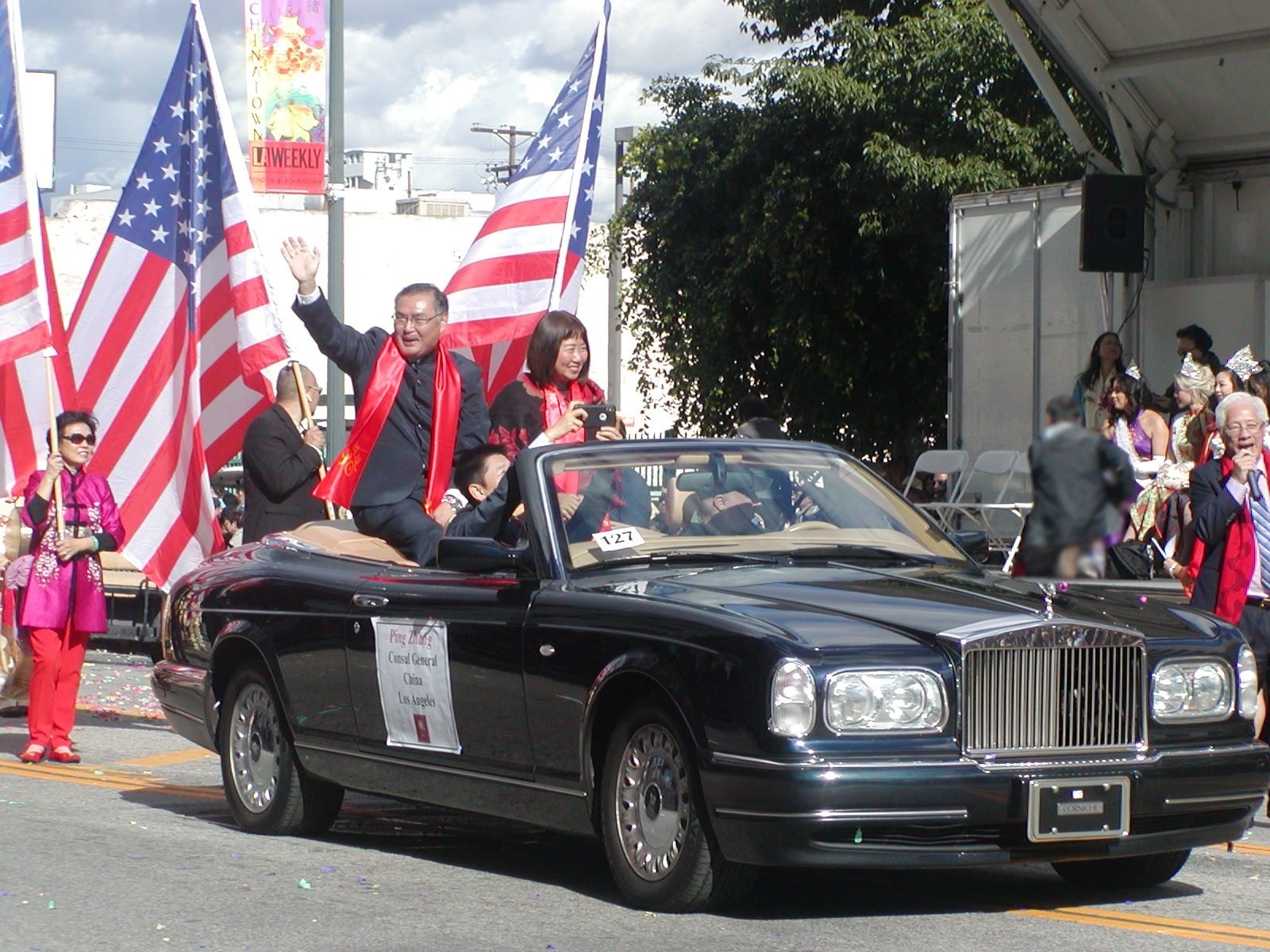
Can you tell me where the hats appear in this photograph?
[698,464,773,501]
[897,476,922,490]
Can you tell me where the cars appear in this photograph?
[92,465,244,645]
[148,435,1270,913]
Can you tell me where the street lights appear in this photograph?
[608,126,645,429]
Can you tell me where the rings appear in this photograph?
[60,553,66,556]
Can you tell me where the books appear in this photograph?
[1151,537,1167,560]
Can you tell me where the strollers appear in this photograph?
[1100,458,1157,580]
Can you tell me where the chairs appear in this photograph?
[903,450,1035,571]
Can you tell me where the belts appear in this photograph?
[1245,596,1270,609]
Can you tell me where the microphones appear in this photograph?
[1242,448,1262,502]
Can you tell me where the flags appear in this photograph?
[0,0,76,503]
[443,0,613,403]
[66,0,298,593]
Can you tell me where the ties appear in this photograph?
[302,430,307,436]
[1249,470,1270,589]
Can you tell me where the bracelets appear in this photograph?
[90,536,99,552]
[1169,562,1177,578]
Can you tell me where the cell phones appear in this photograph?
[573,404,617,429]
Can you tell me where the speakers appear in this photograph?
[1077,172,1146,273]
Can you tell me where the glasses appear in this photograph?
[305,385,325,395]
[61,432,96,445]
[933,480,943,486]
[391,310,447,325]
[1224,421,1265,439]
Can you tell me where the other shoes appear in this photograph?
[48,748,82,763]
[0,706,28,718]
[20,745,47,762]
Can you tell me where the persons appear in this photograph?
[213,477,244,548]
[900,472,947,504]
[1021,394,1132,580]
[1073,332,1126,428]
[1163,537,1205,599]
[1101,324,1270,552]
[444,401,622,558]
[488,311,650,541]
[1190,392,1270,822]
[242,365,341,543]
[677,464,767,535]
[792,482,825,522]
[21,410,126,762]
[738,394,789,519]
[280,236,489,570]
